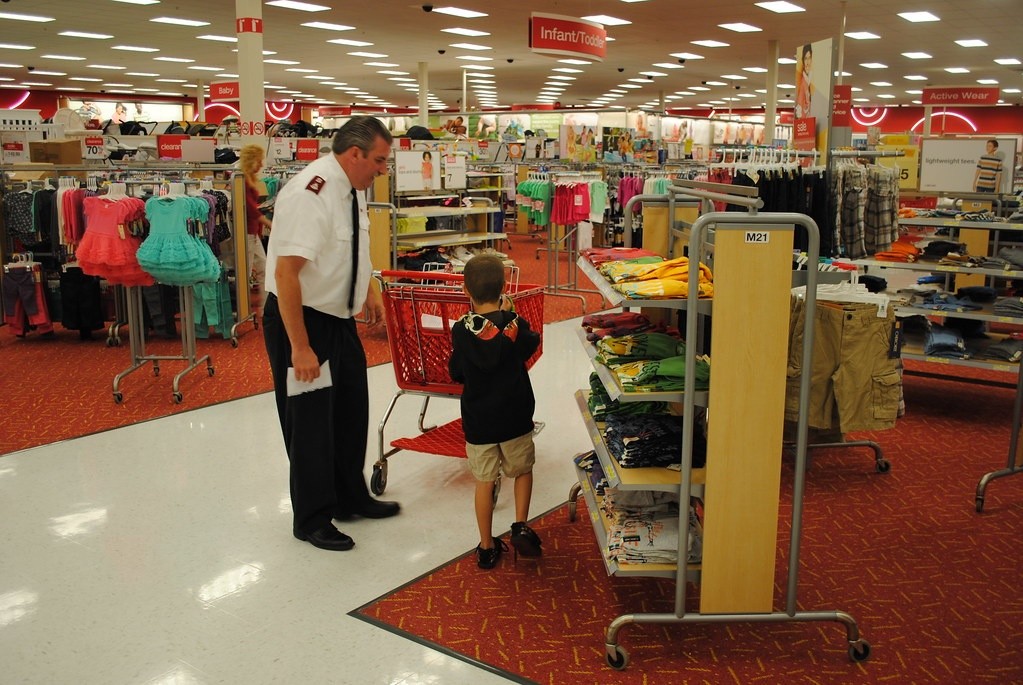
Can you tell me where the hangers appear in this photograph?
[0,163,306,284]
[516,146,903,189]
[789,251,892,320]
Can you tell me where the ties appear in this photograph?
[348,188,360,311]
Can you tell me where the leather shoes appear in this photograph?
[336,496,400,521]
[294,522,355,551]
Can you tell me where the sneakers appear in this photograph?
[510,521,543,564]
[475,537,509,569]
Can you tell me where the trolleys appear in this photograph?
[370,262,545,495]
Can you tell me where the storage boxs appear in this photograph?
[28,139,82,164]
[546,140,560,159]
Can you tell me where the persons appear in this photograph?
[134,102,151,122]
[260,117,401,550]
[565,125,596,163]
[110,102,128,123]
[422,152,432,189]
[679,120,688,139]
[794,43,813,119]
[568,114,585,127]
[443,117,466,135]
[723,124,764,145]
[79,100,104,125]
[239,145,271,320]
[973,139,1003,193]
[634,115,647,138]
[603,128,634,163]
[449,253,542,569]
[474,115,497,136]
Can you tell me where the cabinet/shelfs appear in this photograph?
[572,177,872,672]
[365,196,514,309]
[854,218,1023,374]
